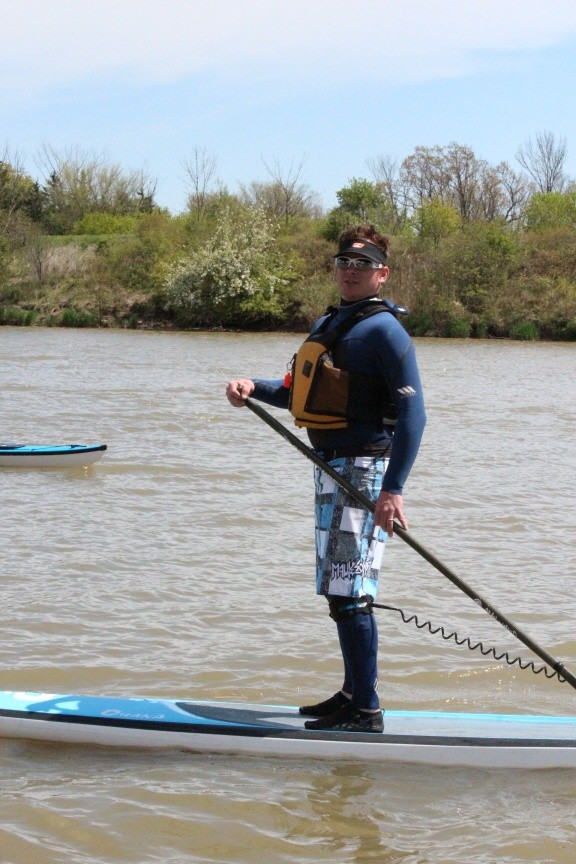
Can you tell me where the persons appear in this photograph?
[226,224,426,733]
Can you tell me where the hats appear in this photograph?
[333,239,388,265]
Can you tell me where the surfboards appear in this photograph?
[0,686,576,772]
[0,442,108,467]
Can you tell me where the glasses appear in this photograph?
[335,255,384,270]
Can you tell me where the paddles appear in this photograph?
[233,387,576,693]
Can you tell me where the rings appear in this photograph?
[387,519,394,521]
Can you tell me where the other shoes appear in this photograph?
[304,701,384,733]
[299,691,352,716]
